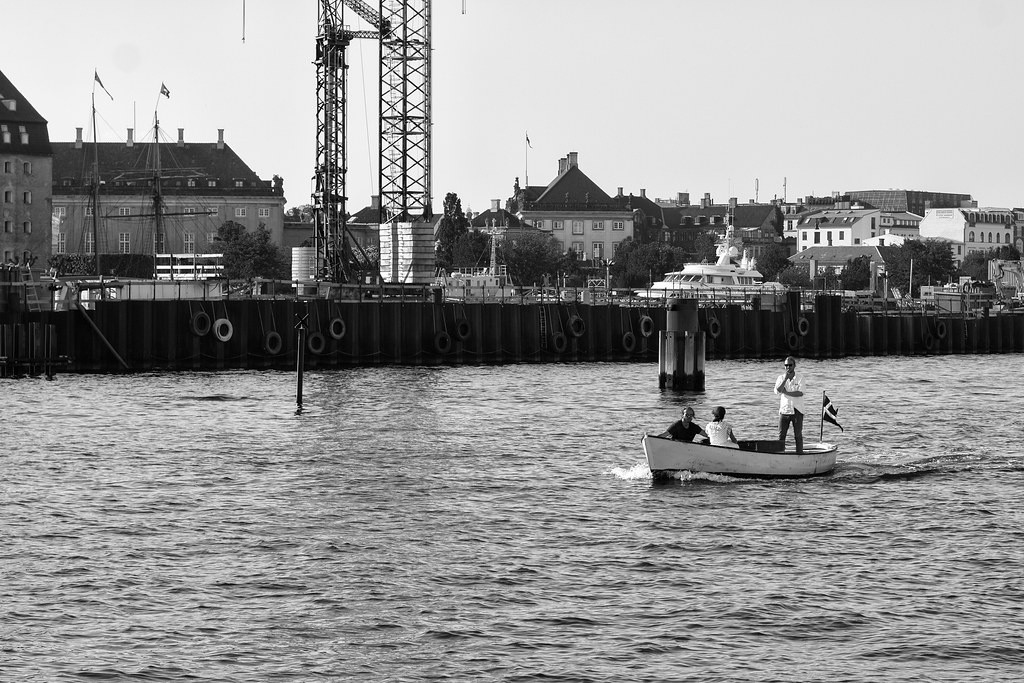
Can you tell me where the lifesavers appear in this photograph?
[214,319,234,341]
[190,312,211,336]
[963,282,971,294]
[308,331,325,353]
[263,331,282,354]
[797,318,810,336]
[937,322,946,339]
[330,318,346,339]
[455,319,471,340]
[566,316,585,338]
[638,316,655,337]
[786,332,798,349]
[708,318,721,337]
[434,331,451,353]
[621,332,636,352]
[552,332,566,351]
[924,334,934,349]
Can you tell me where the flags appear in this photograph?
[160,82,170,99]
[822,394,844,432]
[526,134,532,148]
[94,71,113,100]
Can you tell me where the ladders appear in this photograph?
[19,262,41,313]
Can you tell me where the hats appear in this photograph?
[712,407,726,416]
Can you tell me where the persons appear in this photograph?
[704,406,740,449]
[657,406,708,442]
[773,357,806,454]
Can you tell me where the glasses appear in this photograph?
[785,364,794,366]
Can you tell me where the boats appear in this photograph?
[640,431,839,478]
[629,223,792,307]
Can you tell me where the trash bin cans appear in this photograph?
[432,285,443,303]
[815,294,842,317]
